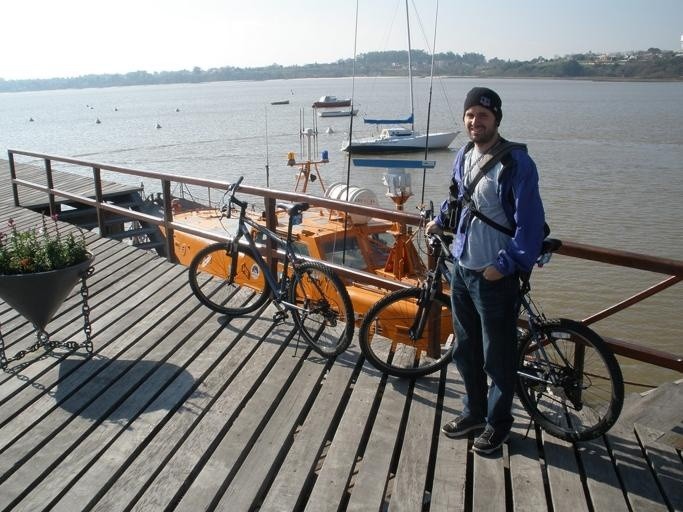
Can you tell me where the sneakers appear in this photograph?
[441,416,487,437]
[472,431,510,455]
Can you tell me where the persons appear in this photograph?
[422,85,544,459]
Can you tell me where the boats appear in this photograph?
[312,95,359,116]
[153,101,456,356]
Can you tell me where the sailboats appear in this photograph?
[342,0,464,154]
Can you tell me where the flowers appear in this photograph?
[0,214,87,270]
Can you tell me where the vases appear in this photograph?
[0,249,96,369]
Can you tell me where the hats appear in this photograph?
[463,87,502,127]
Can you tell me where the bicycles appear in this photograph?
[357,202,622,442]
[186,177,353,358]
